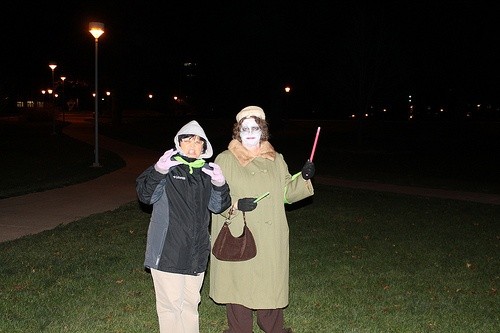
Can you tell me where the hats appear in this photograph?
[235,105,266,121]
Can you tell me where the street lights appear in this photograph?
[284,86,291,129]
[60,76,66,126]
[48,64,58,136]
[88,19,105,167]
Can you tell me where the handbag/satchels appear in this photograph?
[211,205,257,262]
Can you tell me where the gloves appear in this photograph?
[202,163,224,184]
[156,148,182,170]
[238,198,257,211]
[301,160,315,180]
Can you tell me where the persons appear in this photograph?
[138,120,231,333]
[210,106,314,333]
